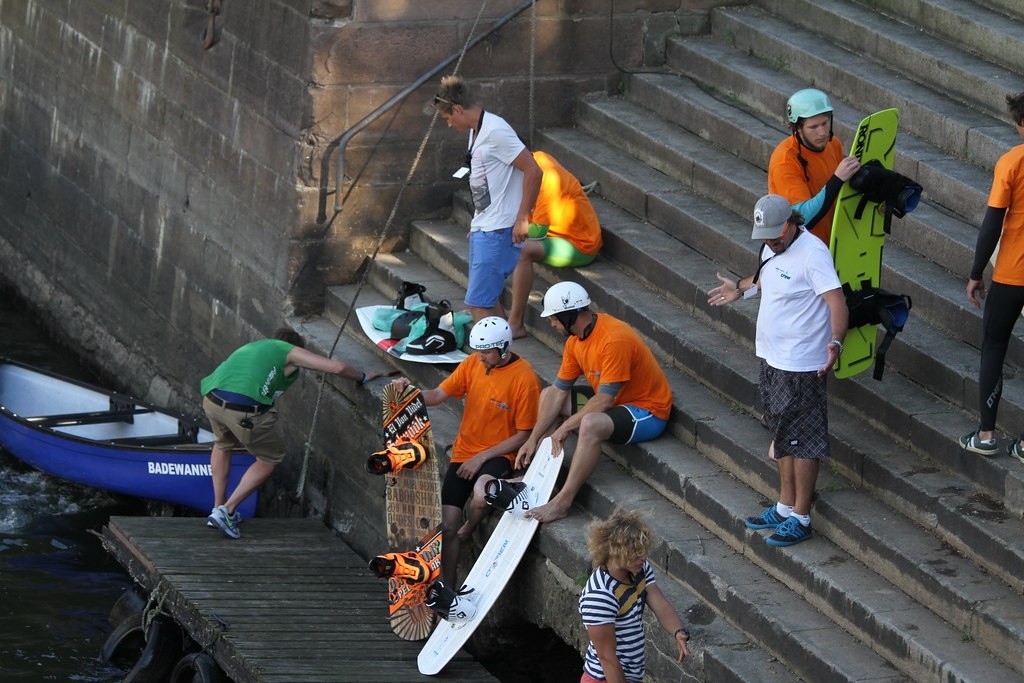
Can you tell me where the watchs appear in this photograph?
[830,340,844,356]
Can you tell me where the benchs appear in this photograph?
[21,393,156,426]
[96,414,200,448]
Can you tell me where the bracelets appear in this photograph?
[356,370,369,385]
[675,628,690,642]
[736,280,744,297]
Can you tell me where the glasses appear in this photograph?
[434,94,455,106]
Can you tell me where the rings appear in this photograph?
[722,296,725,300]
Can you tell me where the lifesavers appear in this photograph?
[169,652,224,683]
[97,609,183,683]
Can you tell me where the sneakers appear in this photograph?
[766,515,812,546]
[845,287,909,333]
[1006,440,1024,463]
[206,509,241,527]
[484,480,538,521]
[424,580,477,629]
[959,430,999,455]
[366,441,430,476]
[850,159,924,218]
[396,282,428,310]
[208,505,240,539]
[406,311,456,355]
[745,502,794,529]
[369,551,431,585]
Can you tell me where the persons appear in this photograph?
[200,327,386,539]
[959,93,1024,465]
[578,507,691,683]
[391,316,539,624]
[432,75,603,338]
[707,194,849,547]
[514,280,673,523]
[767,88,861,248]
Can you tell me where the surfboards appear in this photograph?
[355,304,472,365]
[416,434,567,677]
[826,106,901,381]
[381,376,444,641]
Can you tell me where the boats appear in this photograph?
[1,353,257,520]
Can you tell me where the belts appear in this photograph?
[206,393,270,413]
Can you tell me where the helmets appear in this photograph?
[540,281,591,318]
[469,316,512,350]
[391,311,425,340]
[787,89,835,122]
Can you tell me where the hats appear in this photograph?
[752,194,792,239]
